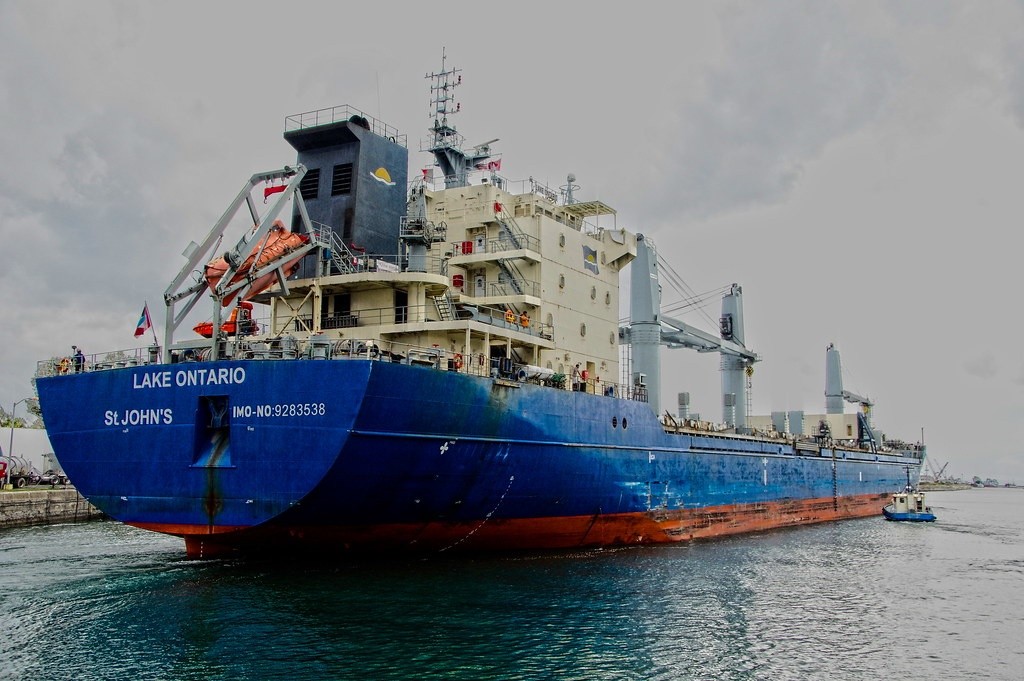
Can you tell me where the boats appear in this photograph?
[882,462,938,524]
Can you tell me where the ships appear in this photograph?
[31,44,926,554]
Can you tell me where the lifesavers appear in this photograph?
[504,310,514,323]
[454,354,463,368]
[60,359,69,372]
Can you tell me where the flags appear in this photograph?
[134,307,152,339]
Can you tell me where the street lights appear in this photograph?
[4,398,40,490]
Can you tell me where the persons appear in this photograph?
[572,364,579,383]
[520,311,530,327]
[70,348,85,372]
